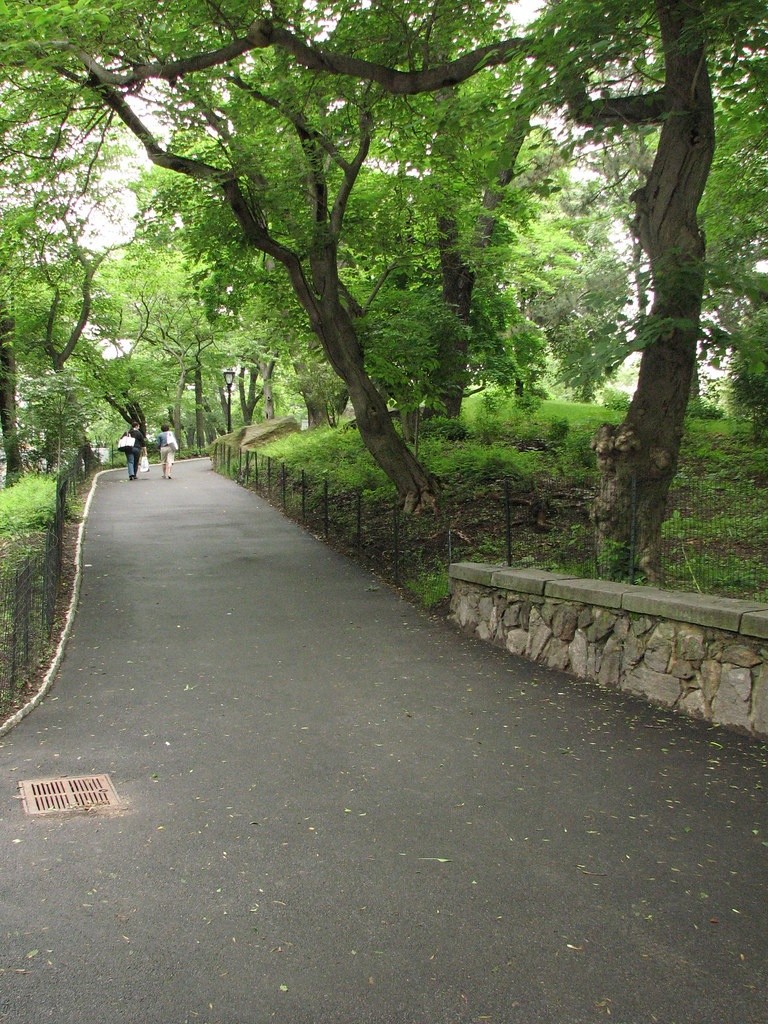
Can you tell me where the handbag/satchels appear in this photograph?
[140,456,149,472]
[167,431,178,452]
[118,432,135,452]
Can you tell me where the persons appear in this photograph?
[120,422,147,480]
[157,425,175,479]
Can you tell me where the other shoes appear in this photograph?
[167,476,171,479]
[161,476,166,478]
[129,476,132,480]
[133,475,137,479]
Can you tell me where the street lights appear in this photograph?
[223,366,235,433]
